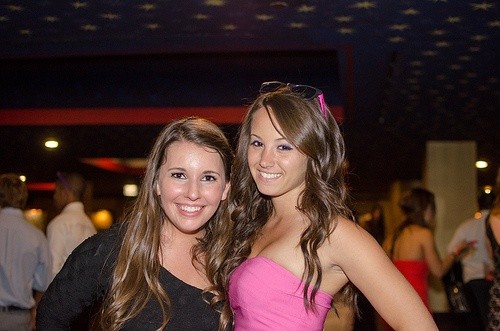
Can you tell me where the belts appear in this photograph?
[1,305,31,312]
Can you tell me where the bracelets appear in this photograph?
[452,248,468,261]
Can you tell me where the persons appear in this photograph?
[46,174,96,288]
[447,191,496,331]
[383,187,479,314]
[484,169,500,331]
[35,115,235,331]
[0,173,52,331]
[205,86,439,331]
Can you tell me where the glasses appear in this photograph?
[259,81,326,119]
